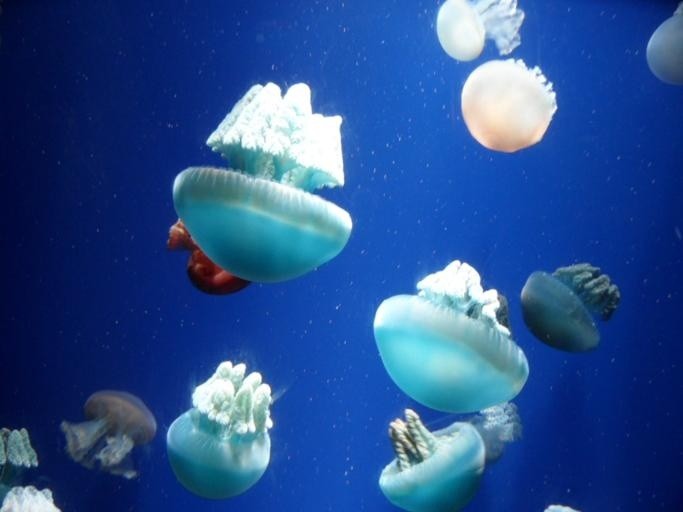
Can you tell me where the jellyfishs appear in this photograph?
[518,260,620,355]
[643,1,681,89]
[0,482,60,512]
[458,55,559,156]
[56,387,158,483]
[435,0,525,65]
[0,423,40,471]
[371,257,531,511]
[161,355,276,500]
[169,81,354,286]
